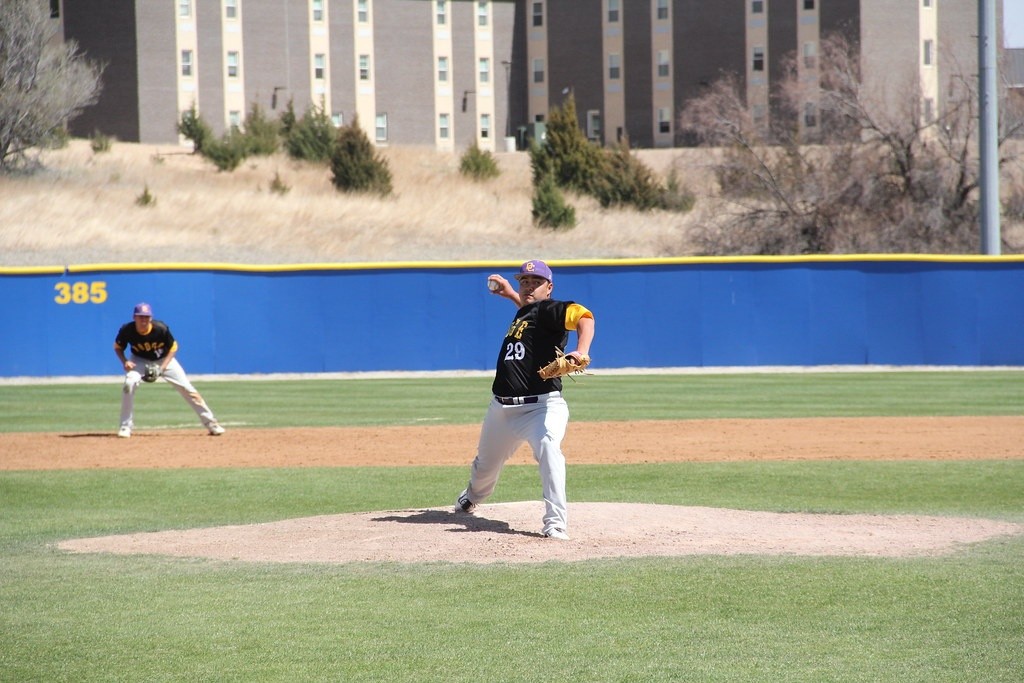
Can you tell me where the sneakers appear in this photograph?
[117,424,131,436]
[207,422,224,434]
[545,528,570,540]
[455,489,481,512]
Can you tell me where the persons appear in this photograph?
[114,303,225,438]
[453,259,595,538]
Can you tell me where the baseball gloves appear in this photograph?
[140,364,159,383]
[537,346,592,382]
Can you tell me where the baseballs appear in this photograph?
[487,278,499,291]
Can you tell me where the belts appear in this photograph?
[494,394,539,405]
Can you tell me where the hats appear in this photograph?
[515,260,552,284]
[133,303,152,318]
